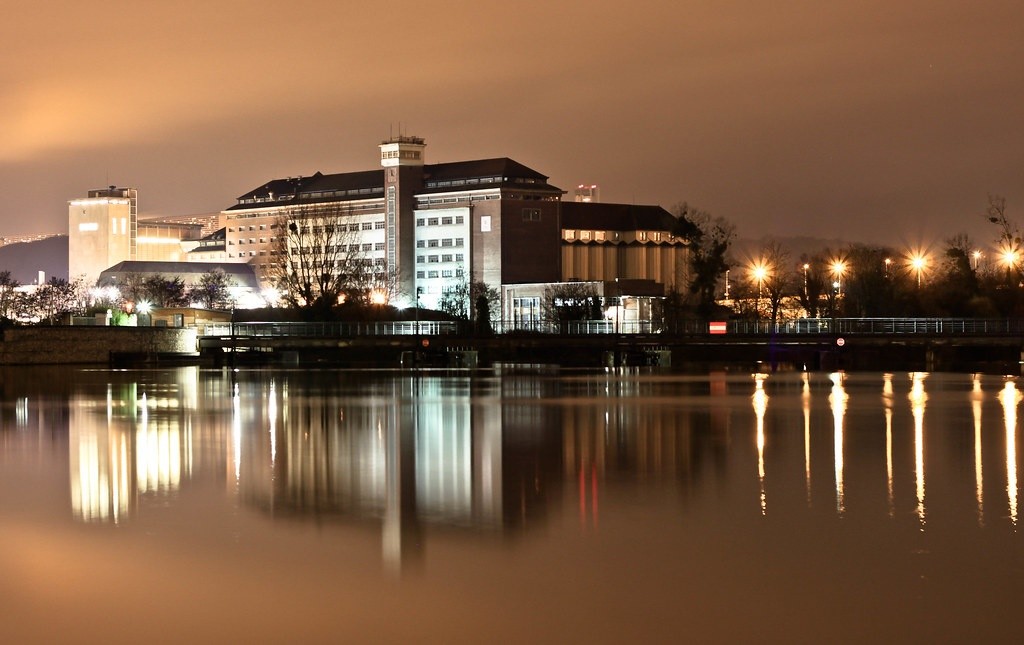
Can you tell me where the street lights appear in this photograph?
[973,251,979,269]
[914,258,924,288]
[802,262,809,294]
[884,258,890,276]
[835,262,844,293]
[755,266,765,299]
